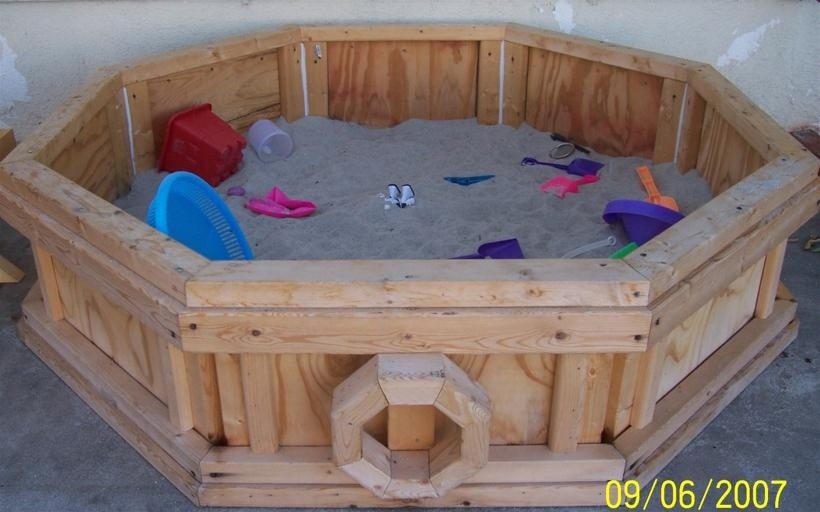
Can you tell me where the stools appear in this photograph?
[1,128,25,284]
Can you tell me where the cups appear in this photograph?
[247,119,294,163]
